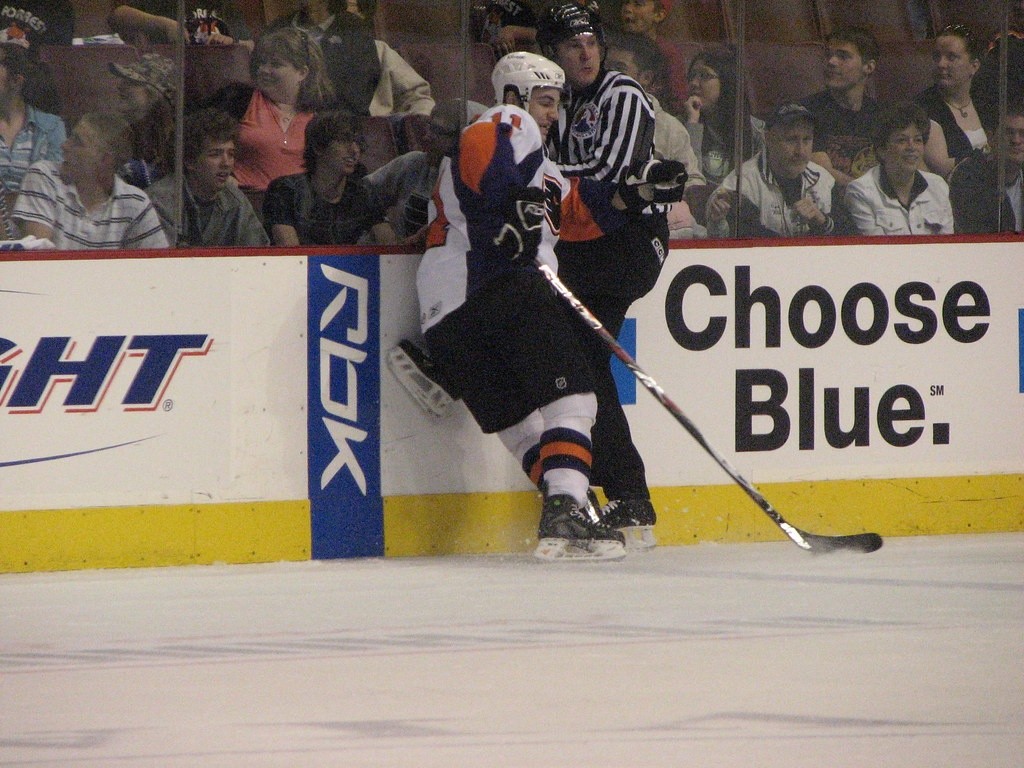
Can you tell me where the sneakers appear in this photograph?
[601,497,660,550]
[389,339,469,417]
[531,480,626,562]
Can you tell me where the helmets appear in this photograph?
[491,51,565,112]
[535,0,609,56]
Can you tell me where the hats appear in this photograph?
[110,53,174,105]
[765,101,818,132]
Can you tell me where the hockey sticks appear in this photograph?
[533,253,883,556]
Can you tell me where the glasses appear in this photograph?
[689,70,719,81]
[945,25,976,47]
[301,31,310,63]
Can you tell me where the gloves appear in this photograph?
[488,187,548,267]
[618,159,688,215]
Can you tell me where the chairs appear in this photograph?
[34,37,940,228]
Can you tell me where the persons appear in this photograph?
[387,0,670,551]
[948,102,1024,233]
[606,0,1024,238]
[706,103,842,237]
[842,101,956,236]
[414,51,627,560]
[0,0,490,249]
[469,0,539,60]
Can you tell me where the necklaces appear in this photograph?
[944,97,971,118]
